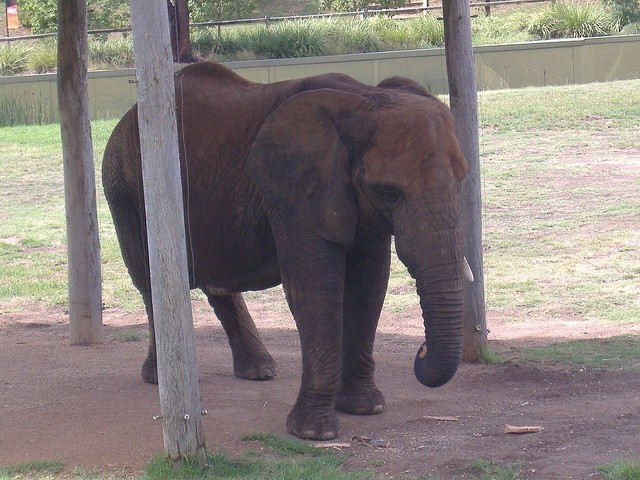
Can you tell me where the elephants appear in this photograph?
[101,62,474,441]
